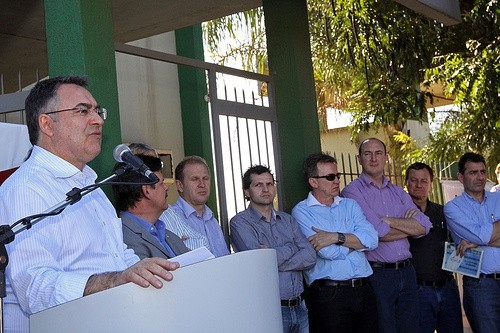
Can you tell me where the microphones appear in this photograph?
[113,145,160,183]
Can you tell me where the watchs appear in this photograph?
[108,153,194,263]
[335,232,346,245]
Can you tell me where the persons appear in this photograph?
[340,137,434,333]
[0,76,179,332]
[167,155,232,258]
[122,142,211,262]
[291,153,382,333]
[488,163,500,192]
[398,162,464,333]
[230,165,316,333]
[442,151,500,333]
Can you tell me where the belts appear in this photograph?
[369,258,410,269]
[281,290,305,307]
[480,272,500,279]
[417,279,444,287]
[313,277,371,286]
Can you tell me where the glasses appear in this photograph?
[313,172,341,180]
[45,106,107,119]
